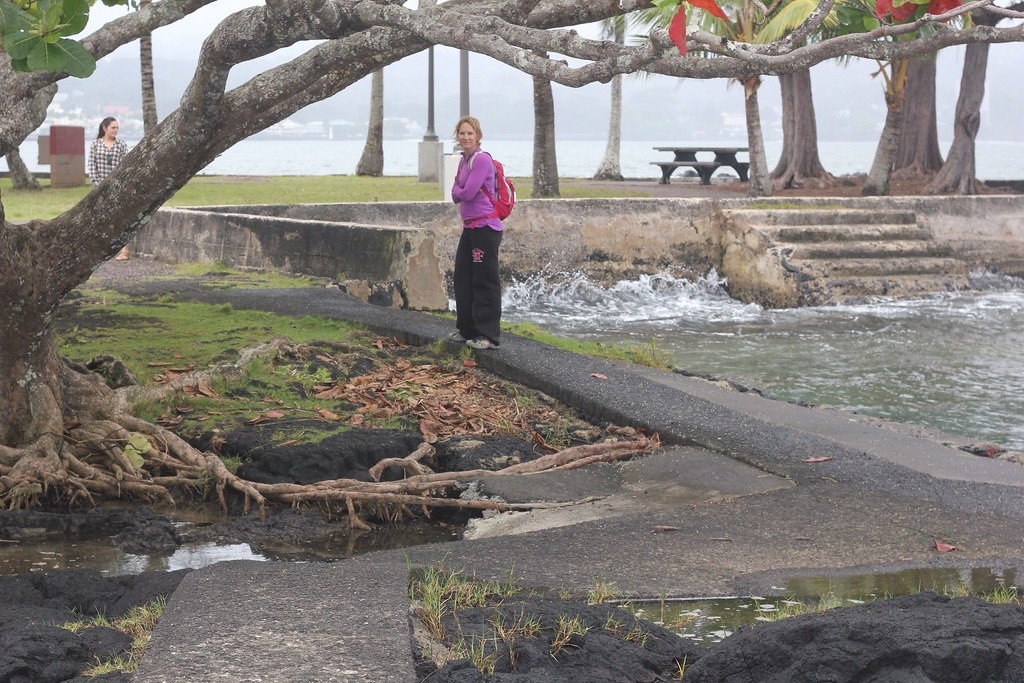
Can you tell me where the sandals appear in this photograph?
[115,250,129,262]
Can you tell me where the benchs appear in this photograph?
[650,148,750,185]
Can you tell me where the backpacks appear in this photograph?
[463,150,517,229]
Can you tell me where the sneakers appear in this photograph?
[448,330,500,350]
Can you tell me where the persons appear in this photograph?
[86,116,130,260]
[452,116,505,352]
